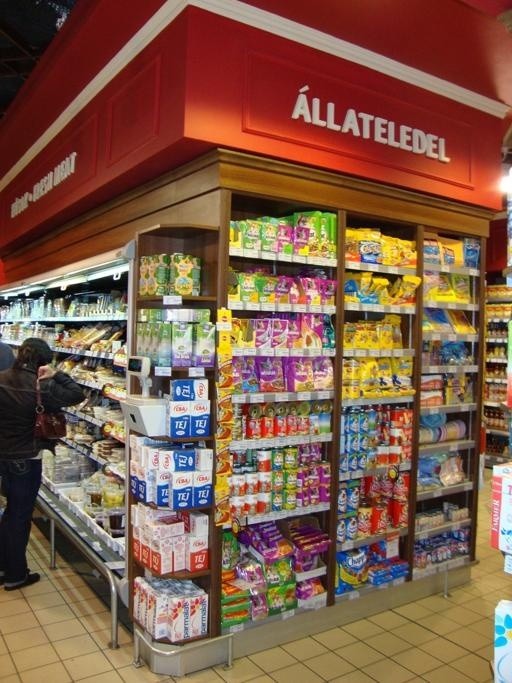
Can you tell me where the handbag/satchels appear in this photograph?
[34,405,66,440]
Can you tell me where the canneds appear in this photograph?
[232,398,413,543]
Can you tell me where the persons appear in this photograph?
[0,333,18,370]
[0,338,85,591]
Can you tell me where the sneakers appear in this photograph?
[0,568,40,591]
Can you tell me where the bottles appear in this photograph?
[484,319,508,456]
